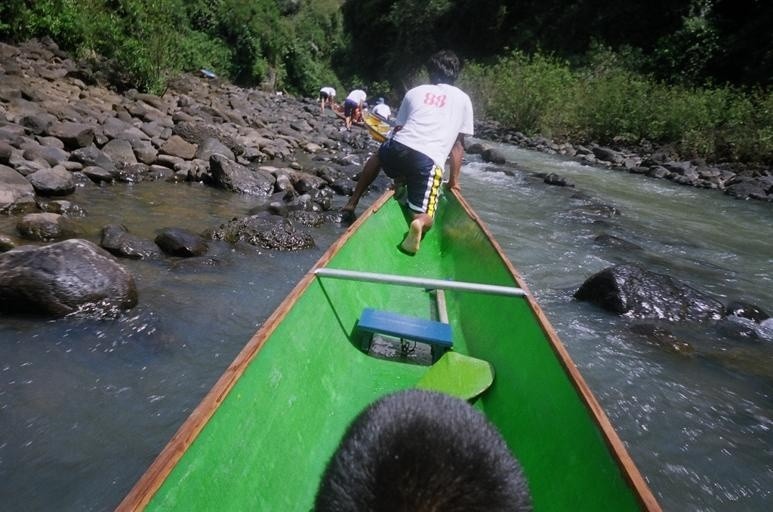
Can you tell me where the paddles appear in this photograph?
[415,279,494,402]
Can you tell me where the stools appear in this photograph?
[354,307,453,365]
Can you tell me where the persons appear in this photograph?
[311,386,532,512]
[339,52,474,255]
[344,86,366,131]
[370,97,392,121]
[319,85,338,113]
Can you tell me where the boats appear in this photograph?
[360,106,393,143]
[330,103,364,126]
[106,181,668,511]
